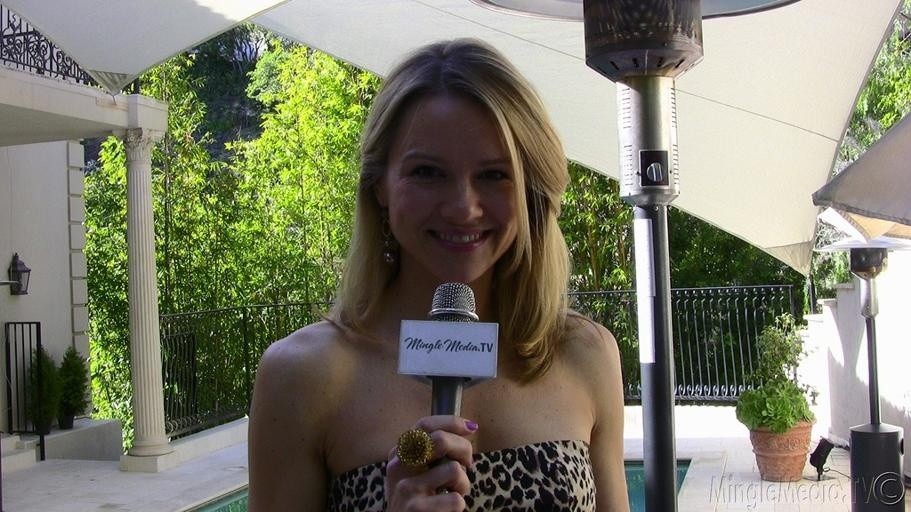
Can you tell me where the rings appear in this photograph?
[396,428,432,468]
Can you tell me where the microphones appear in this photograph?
[396,282,498,493]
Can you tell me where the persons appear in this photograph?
[248,38,630,511]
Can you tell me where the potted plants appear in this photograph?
[734,371,817,482]
[21,343,92,434]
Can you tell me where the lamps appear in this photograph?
[6,251,32,295]
[810,437,835,481]
